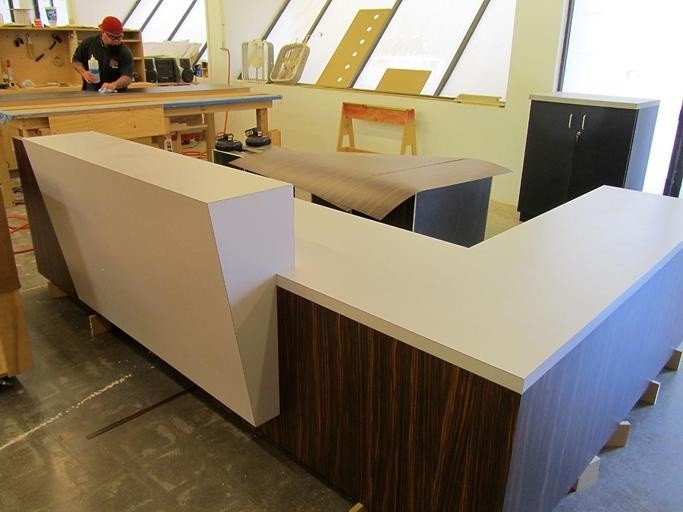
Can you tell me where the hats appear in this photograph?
[98,16,123,34]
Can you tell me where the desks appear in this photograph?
[1,91,285,209]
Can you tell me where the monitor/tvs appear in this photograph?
[156,59,178,83]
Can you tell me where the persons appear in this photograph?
[70,17,134,93]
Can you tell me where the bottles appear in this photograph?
[88,54,101,83]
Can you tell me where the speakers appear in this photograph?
[144,58,158,83]
[179,58,194,83]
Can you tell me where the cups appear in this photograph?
[45,7,58,27]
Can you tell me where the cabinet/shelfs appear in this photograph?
[515,92,659,221]
[0,26,146,89]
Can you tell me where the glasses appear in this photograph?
[105,32,123,41]
